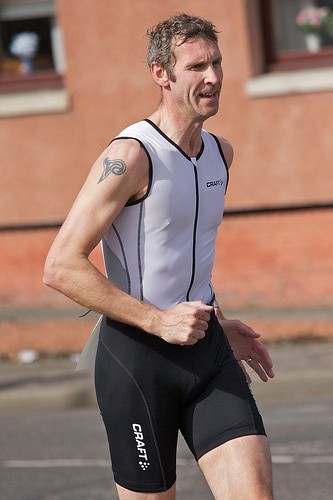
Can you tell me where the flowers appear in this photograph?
[295,6,329,32]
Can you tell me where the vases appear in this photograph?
[306,35,320,53]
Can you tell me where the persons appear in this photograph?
[42,13,274,500]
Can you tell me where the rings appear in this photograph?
[247,358,252,364]
